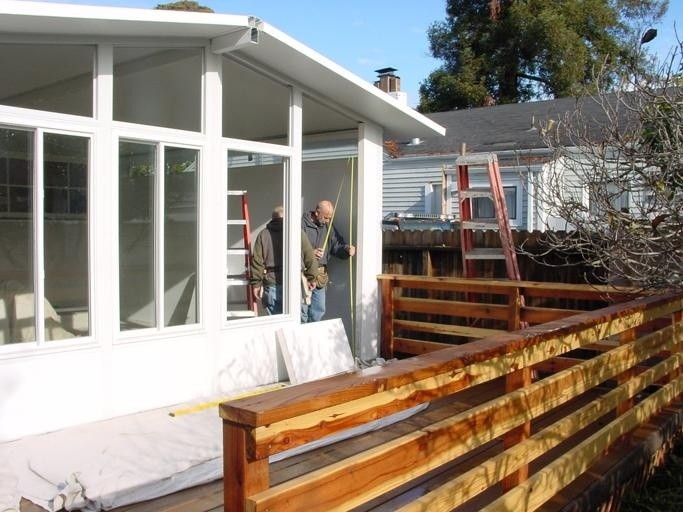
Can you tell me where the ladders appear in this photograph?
[227,189,258,320]
[456,153,527,334]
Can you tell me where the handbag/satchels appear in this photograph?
[316,267,329,288]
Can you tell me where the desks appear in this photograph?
[0,279,52,346]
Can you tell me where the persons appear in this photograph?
[248,206,318,323]
[300,201,355,323]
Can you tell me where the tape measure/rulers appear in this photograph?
[318,157,355,366]
